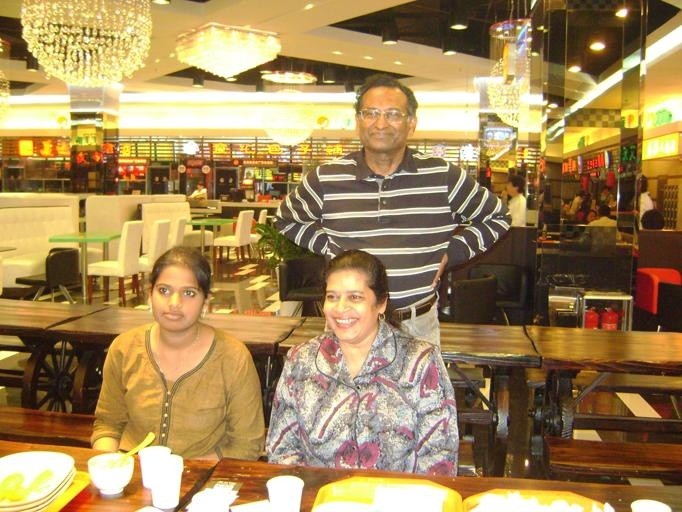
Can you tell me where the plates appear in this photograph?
[0,450,78,511]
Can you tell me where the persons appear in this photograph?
[639,173,653,230]
[273,71,512,348]
[560,184,622,256]
[187,180,207,199]
[264,250,459,478]
[500,173,526,229]
[640,208,671,231]
[90,246,263,463]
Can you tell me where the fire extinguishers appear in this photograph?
[585,300,599,328]
[599,300,618,330]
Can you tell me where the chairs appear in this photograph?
[279,260,530,325]
[0,193,267,307]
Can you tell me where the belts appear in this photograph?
[393,295,437,322]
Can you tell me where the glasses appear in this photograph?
[359,109,409,122]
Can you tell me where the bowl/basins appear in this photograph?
[87,453,134,497]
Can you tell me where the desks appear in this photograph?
[0,299,109,412]
[279,318,542,476]
[46,307,306,426]
[526,325,682,440]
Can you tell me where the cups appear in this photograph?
[266,476,305,512]
[150,454,183,510]
[631,497,672,512]
[139,446,172,490]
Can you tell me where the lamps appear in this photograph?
[381,22,399,44]
[321,66,335,83]
[176,22,280,79]
[441,32,458,55]
[261,59,318,85]
[261,89,315,147]
[20,0,152,87]
[448,4,469,29]
[487,0,533,127]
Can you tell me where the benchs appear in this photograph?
[526,368,682,409]
[0,406,97,445]
[543,435,682,481]
